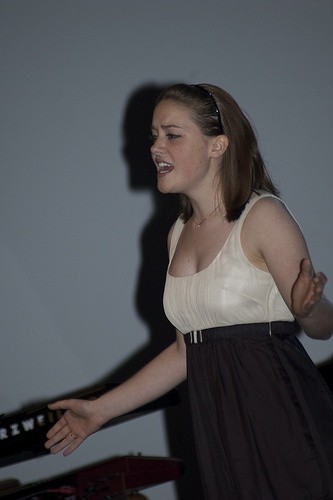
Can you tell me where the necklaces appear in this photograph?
[191,199,225,233]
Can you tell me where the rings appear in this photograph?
[68,433,77,439]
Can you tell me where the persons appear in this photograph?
[43,83,332,500]
